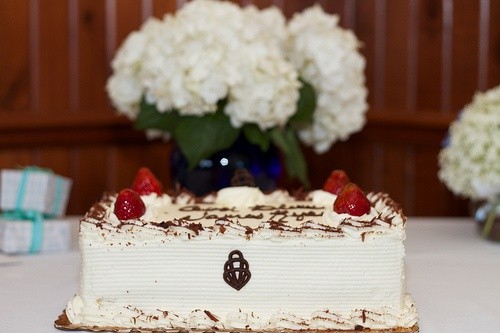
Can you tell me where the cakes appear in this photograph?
[64,167,419,330]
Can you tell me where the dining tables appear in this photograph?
[0,218,499,333]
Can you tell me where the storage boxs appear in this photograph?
[0,169,70,255]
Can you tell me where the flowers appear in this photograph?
[106,0,369,188]
[438,86,500,242]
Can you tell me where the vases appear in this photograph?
[168,146,278,197]
